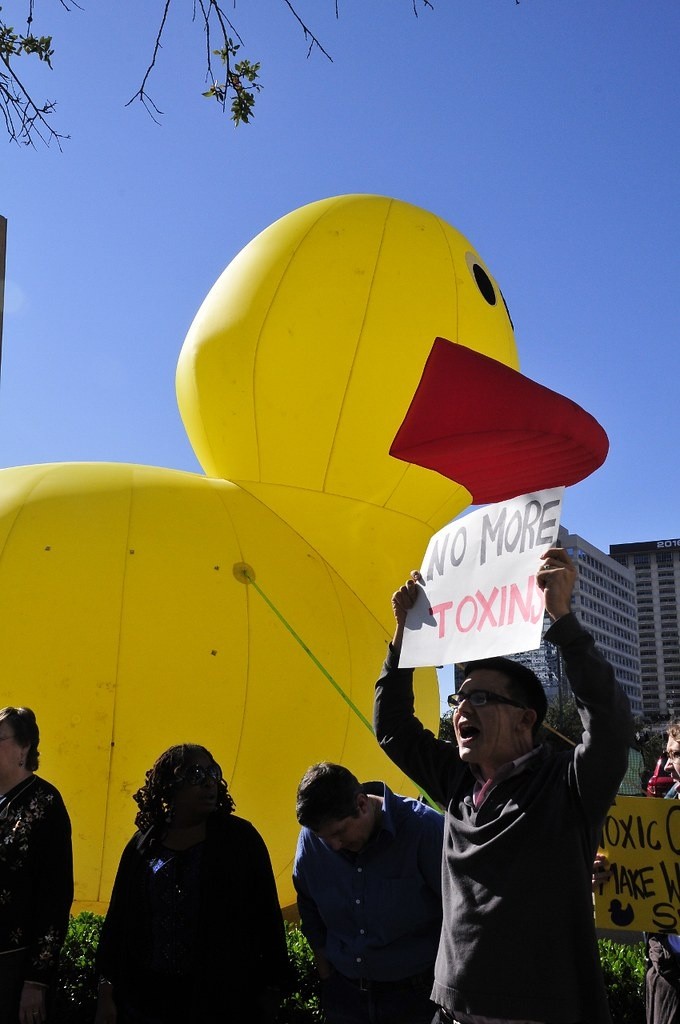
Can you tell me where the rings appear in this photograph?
[545,565,550,569]
[32,1009,38,1014]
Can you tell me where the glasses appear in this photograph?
[180,764,222,785]
[447,690,528,711]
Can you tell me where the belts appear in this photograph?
[440,1008,462,1024]
[338,965,433,992]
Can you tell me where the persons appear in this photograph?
[373,540,635,1024]
[590,724,680,1024]
[0,706,74,1024]
[292,762,446,1024]
[74,743,291,1024]
